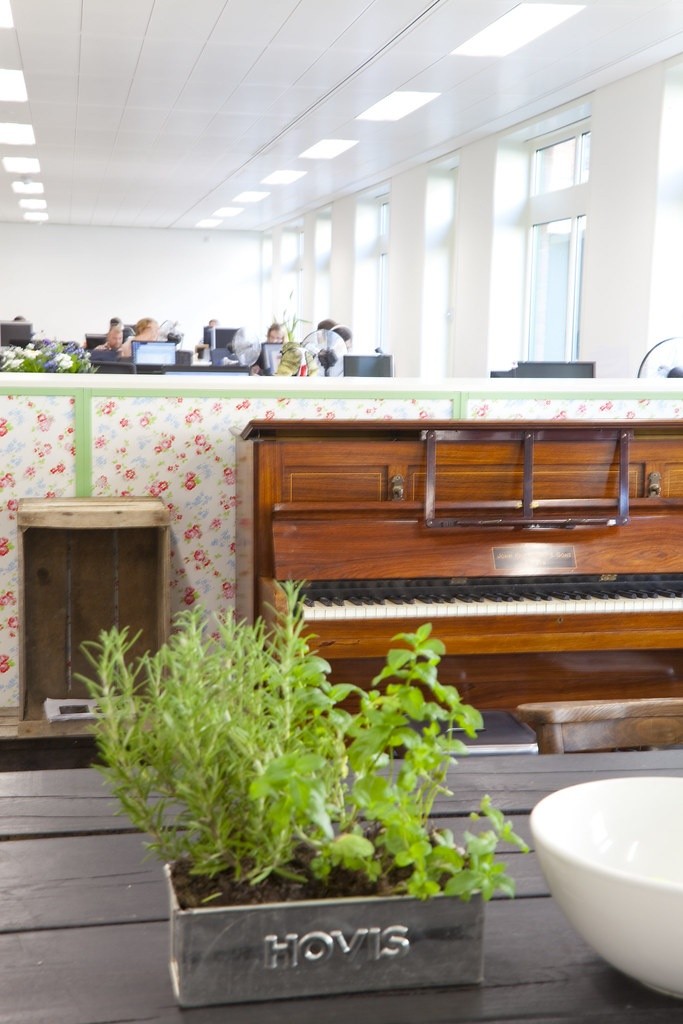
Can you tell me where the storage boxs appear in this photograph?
[16,496,174,740]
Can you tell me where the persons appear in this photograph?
[208,320,217,326]
[94,319,123,351]
[250,324,281,376]
[317,320,350,347]
[118,318,158,357]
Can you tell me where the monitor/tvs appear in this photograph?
[0,322,34,347]
[84,328,593,380]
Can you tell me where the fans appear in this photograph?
[156,319,184,352]
[227,325,262,367]
[300,329,347,377]
[637,337,683,378]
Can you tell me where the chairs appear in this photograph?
[515,696,683,754]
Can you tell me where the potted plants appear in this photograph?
[72,571,533,1009]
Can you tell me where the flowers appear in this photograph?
[0,336,102,374]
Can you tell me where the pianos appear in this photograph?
[235,419,683,751]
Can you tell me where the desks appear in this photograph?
[0,749,683,1024]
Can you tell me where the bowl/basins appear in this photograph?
[527,776,683,1007]
